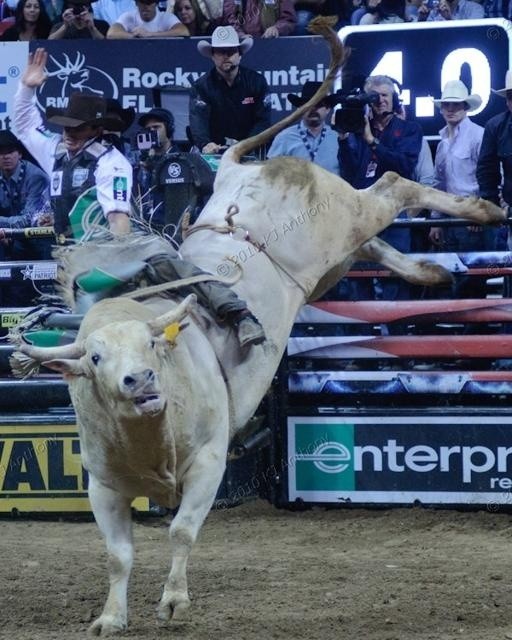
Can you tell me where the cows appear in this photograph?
[18,17,506,639]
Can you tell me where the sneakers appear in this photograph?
[235,315,267,349]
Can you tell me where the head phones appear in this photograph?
[154,110,175,138]
[359,76,402,110]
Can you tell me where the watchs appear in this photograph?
[368,138,380,147]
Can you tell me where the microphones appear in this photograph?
[383,108,395,117]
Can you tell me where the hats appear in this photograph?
[491,70,512,99]
[432,80,482,112]
[137,108,175,138]
[287,80,339,110]
[196,25,253,58]
[0,129,26,154]
[45,92,135,133]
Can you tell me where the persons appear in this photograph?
[1,1,511,42]
[189,26,270,206]
[394,102,438,371]
[429,79,494,370]
[10,47,264,347]
[265,81,343,371]
[37,97,150,227]
[475,69,512,372]
[138,108,213,251]
[330,75,423,370]
[0,129,54,261]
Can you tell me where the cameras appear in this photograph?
[427,0,440,8]
[73,6,85,14]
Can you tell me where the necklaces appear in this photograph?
[165,145,171,152]
